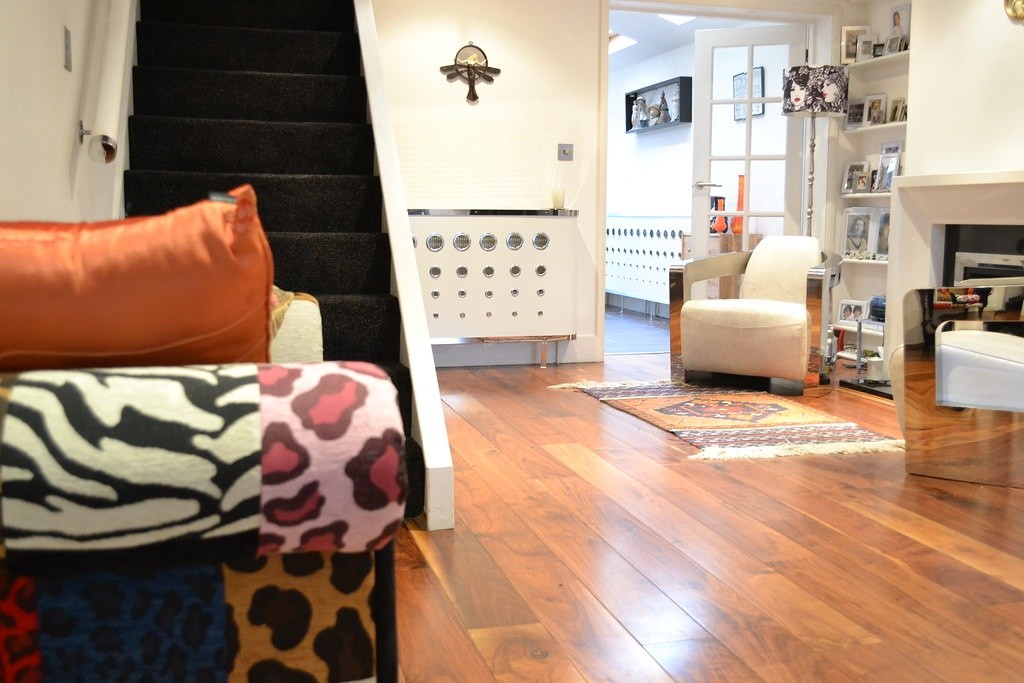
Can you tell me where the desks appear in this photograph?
[409,208,579,369]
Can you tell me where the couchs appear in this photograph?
[890,277,1024,489]
[0,287,408,682]
[669,234,843,398]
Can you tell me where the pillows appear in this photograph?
[0,184,273,373]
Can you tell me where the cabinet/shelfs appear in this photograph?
[625,76,692,134]
[834,49,910,368]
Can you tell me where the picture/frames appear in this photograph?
[839,207,890,262]
[841,142,904,194]
[841,3,911,64]
[732,67,764,121]
[844,93,908,128]
[836,299,870,327]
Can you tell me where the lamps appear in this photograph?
[783,65,849,238]
